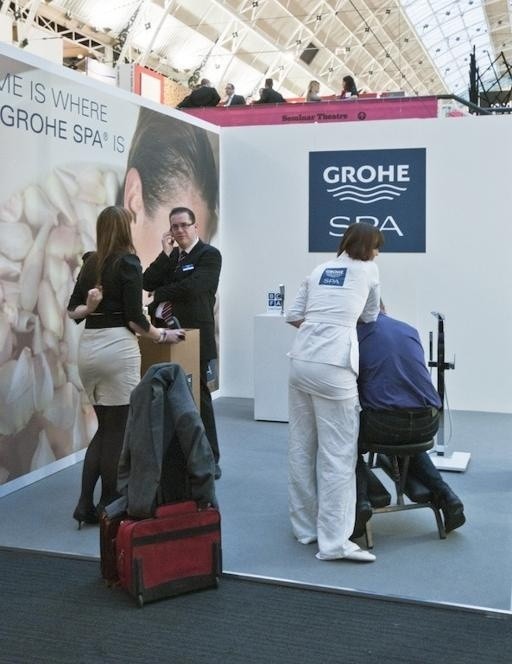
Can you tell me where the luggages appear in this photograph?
[115,499,221,610]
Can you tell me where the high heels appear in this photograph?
[73,500,107,529]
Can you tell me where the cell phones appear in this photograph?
[178,335,185,340]
[170,228,174,243]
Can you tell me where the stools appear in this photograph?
[357,440,447,550]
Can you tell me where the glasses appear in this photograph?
[171,221,195,229]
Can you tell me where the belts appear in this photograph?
[376,405,432,419]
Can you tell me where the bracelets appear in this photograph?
[153,328,168,345]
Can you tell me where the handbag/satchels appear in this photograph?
[99,508,127,587]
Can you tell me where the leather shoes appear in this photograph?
[214,463,222,480]
[344,548,376,562]
[352,502,373,538]
[443,501,465,534]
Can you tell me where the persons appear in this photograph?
[0,109,220,484]
[350,299,465,539]
[175,85,202,108]
[284,222,383,562]
[253,77,287,104]
[259,88,264,95]
[189,79,220,107]
[142,207,221,479]
[72,205,181,530]
[339,76,359,98]
[307,81,320,102]
[222,83,246,107]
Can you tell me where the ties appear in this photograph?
[161,251,188,322]
